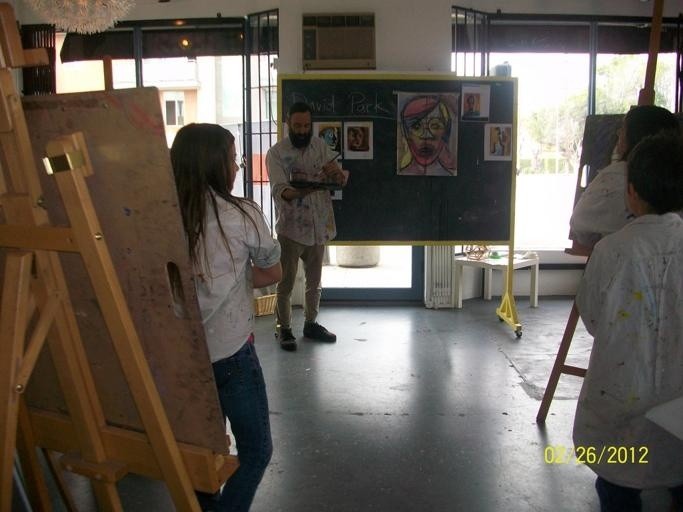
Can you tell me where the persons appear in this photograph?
[571,133,683,512]
[570,103,681,247]
[264,103,347,351]
[168,122,282,512]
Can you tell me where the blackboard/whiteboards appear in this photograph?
[277,72,518,246]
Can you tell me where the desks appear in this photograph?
[455,253,538,310]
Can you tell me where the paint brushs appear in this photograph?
[313,152,341,177]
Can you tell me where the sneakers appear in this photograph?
[302,324,338,344]
[272,327,299,352]
